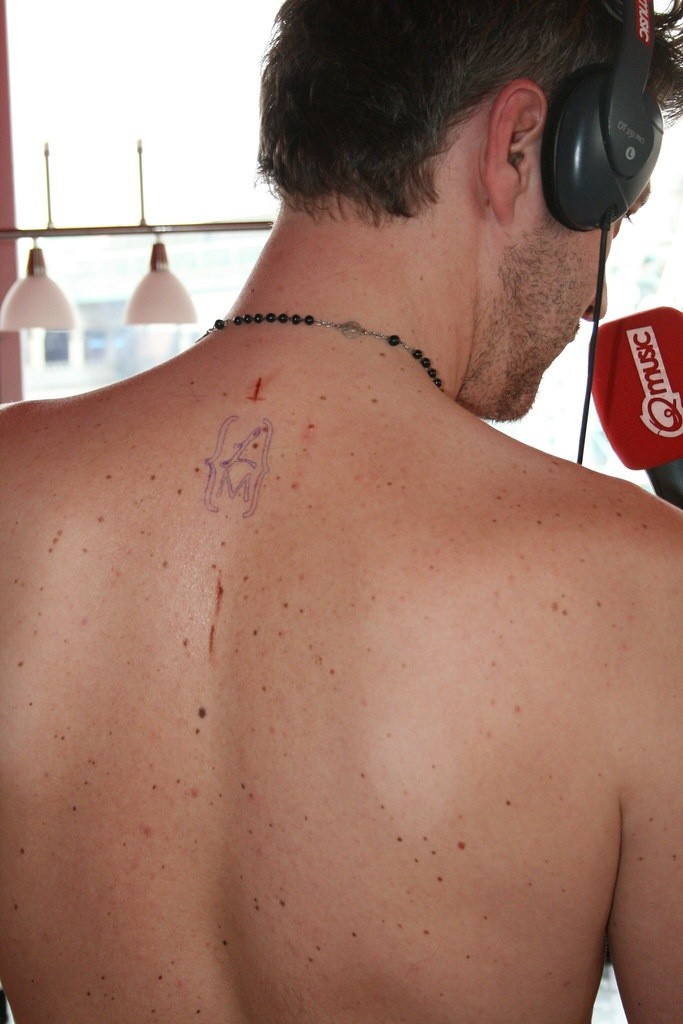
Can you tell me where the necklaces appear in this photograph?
[196,313,445,393]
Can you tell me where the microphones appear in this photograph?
[590,304,682,510]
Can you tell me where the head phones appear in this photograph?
[540,0,663,232]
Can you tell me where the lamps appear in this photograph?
[120,232,200,326]
[0,236,76,333]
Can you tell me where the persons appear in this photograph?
[0,1,683,1024]
